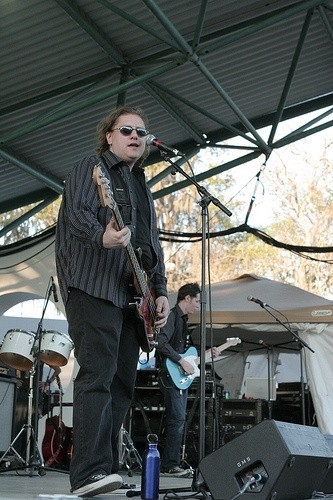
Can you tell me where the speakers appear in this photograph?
[199,398,333,500]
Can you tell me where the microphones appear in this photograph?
[259,340,271,350]
[51,276,58,302]
[247,295,269,307]
[146,134,183,157]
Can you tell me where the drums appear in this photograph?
[0,328,36,371]
[34,329,75,367]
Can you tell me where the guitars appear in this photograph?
[164,337,242,390]
[41,374,67,468]
[92,165,161,353]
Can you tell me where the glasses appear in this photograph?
[107,126,151,136]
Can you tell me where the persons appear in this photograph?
[37,363,61,465]
[156,283,219,474]
[54,107,171,497]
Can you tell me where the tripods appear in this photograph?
[0,277,53,476]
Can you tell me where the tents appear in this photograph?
[166,273,333,434]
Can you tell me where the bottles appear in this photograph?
[141,434,160,500]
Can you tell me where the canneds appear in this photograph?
[224,391,231,400]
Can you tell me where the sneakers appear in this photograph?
[159,467,190,477]
[71,473,122,497]
